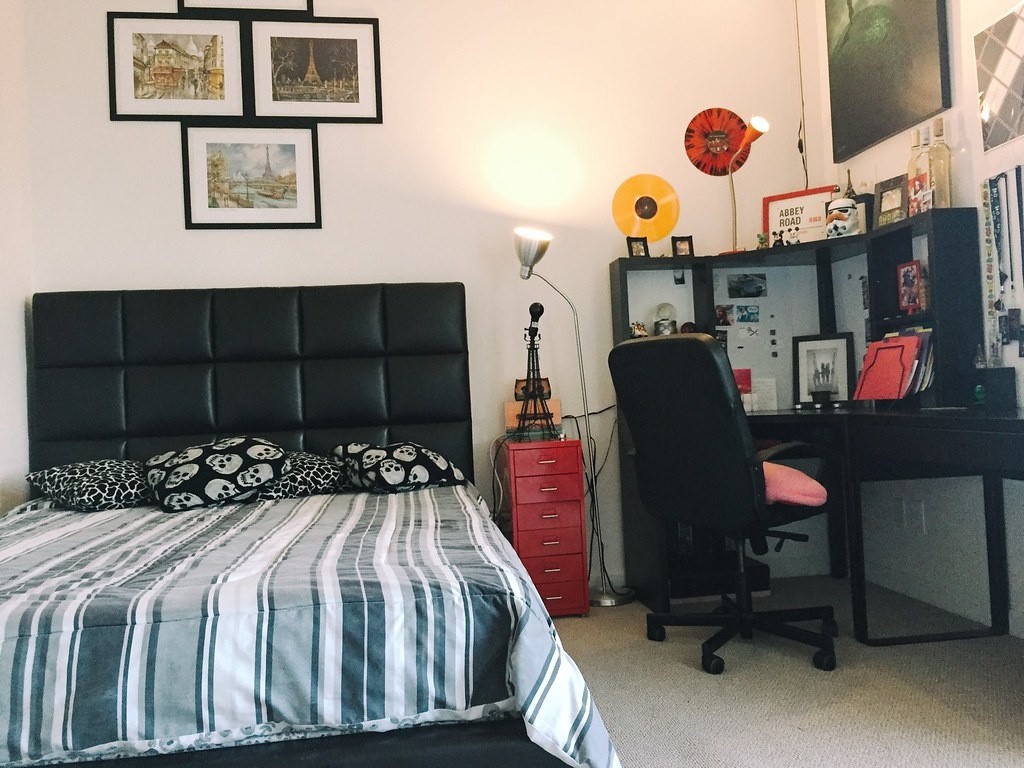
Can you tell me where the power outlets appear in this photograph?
[892,497,907,528]
[911,500,927,536]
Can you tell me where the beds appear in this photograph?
[0,282,622,768]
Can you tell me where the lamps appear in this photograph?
[722,115,769,256]
[514,224,633,609]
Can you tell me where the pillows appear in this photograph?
[327,440,468,496]
[252,451,351,502]
[143,434,293,514]
[25,459,155,513]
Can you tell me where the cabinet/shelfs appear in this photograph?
[609,208,987,412]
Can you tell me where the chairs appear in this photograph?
[607,333,839,674]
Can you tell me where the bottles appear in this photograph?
[858,181,868,195]
[914,126,931,177]
[974,343,987,370]
[988,341,1002,368]
[831,185,841,201]
[930,117,952,209]
[907,128,921,180]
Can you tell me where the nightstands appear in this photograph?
[494,437,591,619]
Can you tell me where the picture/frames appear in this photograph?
[792,331,857,407]
[249,14,383,125]
[670,235,695,258]
[872,172,910,230]
[625,236,650,258]
[106,11,247,121]
[177,0,315,17]
[896,259,921,314]
[181,119,324,231]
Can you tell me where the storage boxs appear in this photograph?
[504,398,562,436]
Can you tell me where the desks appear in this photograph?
[746,403,1024,649]
